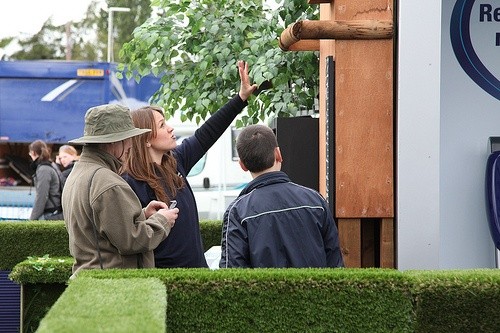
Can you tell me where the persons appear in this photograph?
[119,60,258,268]
[29,139,65,220]
[61,103,179,279]
[55,145,80,178]
[219,125,344,268]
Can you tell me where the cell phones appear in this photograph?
[169,201,177,209]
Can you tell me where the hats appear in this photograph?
[59,145,76,156]
[68,105,152,143]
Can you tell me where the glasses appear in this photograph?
[29,152,32,156]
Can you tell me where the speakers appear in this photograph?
[273,115,319,193]
